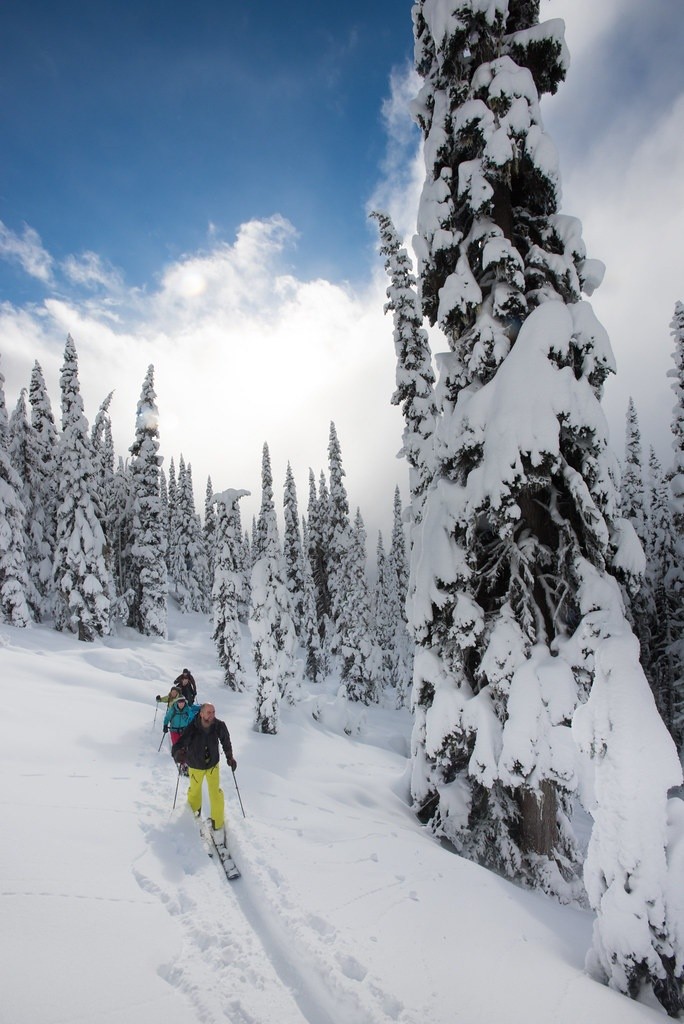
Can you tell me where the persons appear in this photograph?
[174,668,197,696]
[163,697,194,770]
[175,675,195,707]
[172,702,238,832]
[156,687,189,712]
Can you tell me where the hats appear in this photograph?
[183,669,188,673]
[176,696,185,703]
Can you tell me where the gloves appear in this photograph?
[227,757,237,771]
[156,695,161,702]
[163,725,168,733]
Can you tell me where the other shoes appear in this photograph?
[182,766,189,776]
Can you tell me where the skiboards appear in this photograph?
[177,763,189,778]
[193,814,241,881]
